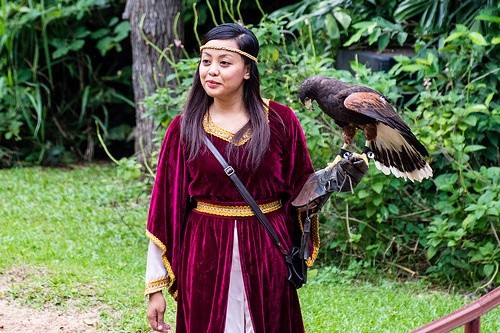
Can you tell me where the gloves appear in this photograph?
[292,158,367,213]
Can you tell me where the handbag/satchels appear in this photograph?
[284,246,307,289]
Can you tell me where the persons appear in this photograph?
[144,24,370,333]
